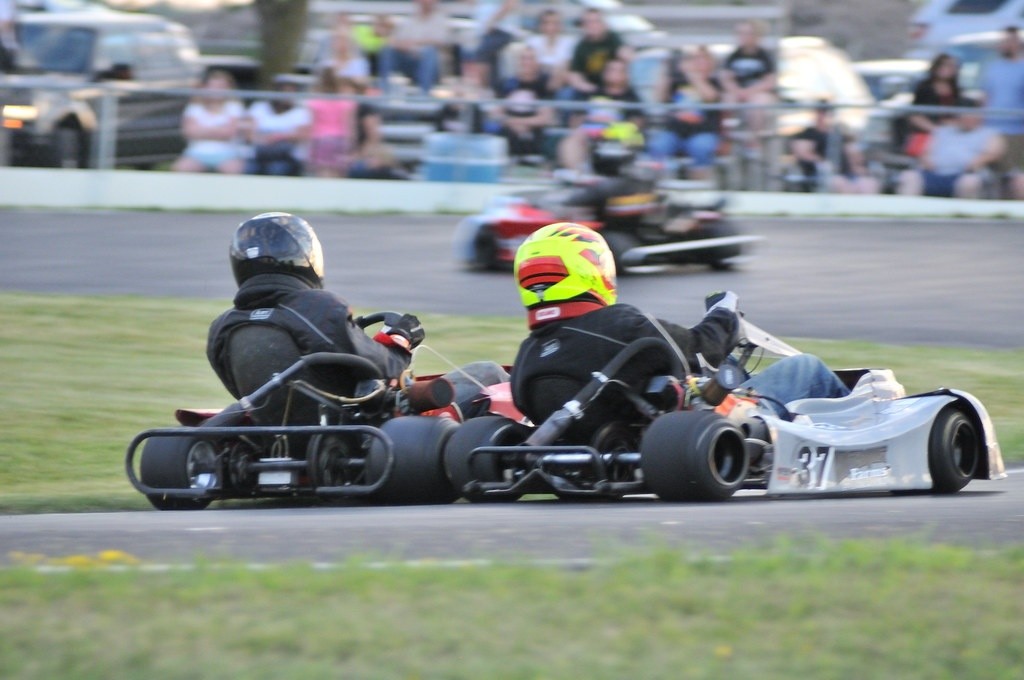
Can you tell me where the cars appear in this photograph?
[907,0,1024,61]
[857,57,934,112]
[629,36,894,163]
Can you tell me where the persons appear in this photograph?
[171,1,1024,237]
[205,210,512,406]
[513,222,852,413]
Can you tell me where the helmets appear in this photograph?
[230,211,325,280]
[603,122,645,147]
[513,221,618,308]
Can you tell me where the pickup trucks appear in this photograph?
[0,11,262,168]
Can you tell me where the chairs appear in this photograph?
[222,320,347,426]
[518,370,642,445]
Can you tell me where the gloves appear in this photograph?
[706,290,739,316]
[372,313,424,352]
